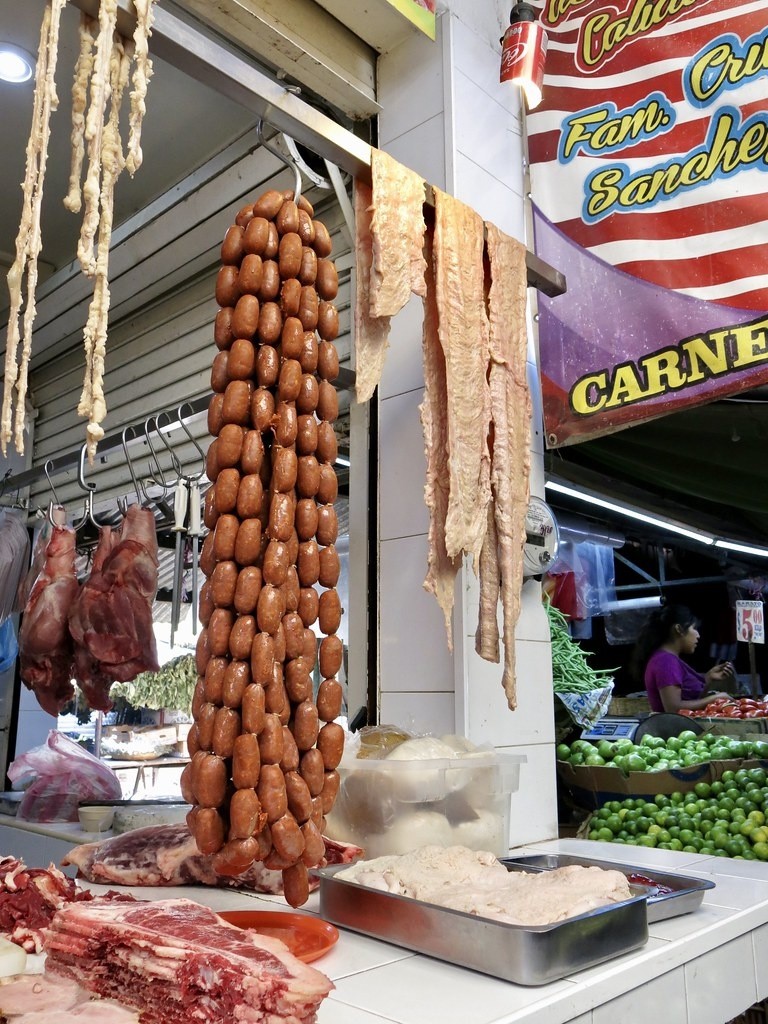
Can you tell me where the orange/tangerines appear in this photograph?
[588,768,768,862]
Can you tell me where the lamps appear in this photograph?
[544,470,768,558]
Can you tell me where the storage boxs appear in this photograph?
[321,751,529,860]
[557,696,768,797]
[105,722,192,759]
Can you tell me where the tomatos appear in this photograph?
[676,695,768,719]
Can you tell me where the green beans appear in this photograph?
[541,590,622,695]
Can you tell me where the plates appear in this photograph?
[216,909,340,964]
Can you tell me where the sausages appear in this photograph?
[178,188,343,910]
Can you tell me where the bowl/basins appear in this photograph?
[78,805,115,832]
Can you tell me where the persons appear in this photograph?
[637,600,735,713]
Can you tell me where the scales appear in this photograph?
[579,715,647,742]
